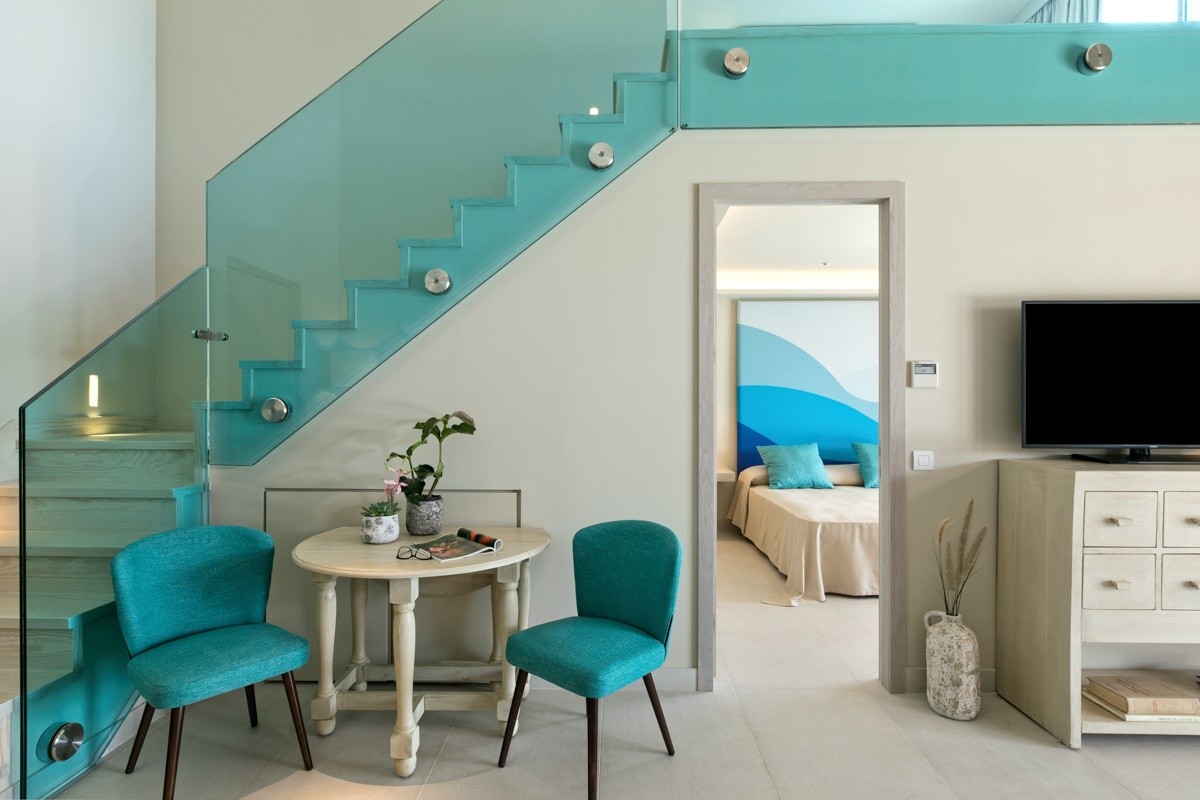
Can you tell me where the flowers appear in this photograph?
[358,457,418,517]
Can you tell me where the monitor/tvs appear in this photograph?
[1020,300,1200,465]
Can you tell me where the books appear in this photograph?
[408,528,504,562]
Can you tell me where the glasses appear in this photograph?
[397,538,448,560]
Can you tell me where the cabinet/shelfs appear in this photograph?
[996,457,1200,750]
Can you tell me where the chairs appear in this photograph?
[496,519,683,800]
[110,524,315,800]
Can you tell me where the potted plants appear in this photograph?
[383,410,476,536]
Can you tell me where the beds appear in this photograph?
[725,463,880,607]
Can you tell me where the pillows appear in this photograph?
[850,441,879,489]
[756,442,835,490]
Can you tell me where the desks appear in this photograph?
[291,524,551,778]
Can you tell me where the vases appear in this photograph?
[360,513,399,544]
[924,610,982,721]
